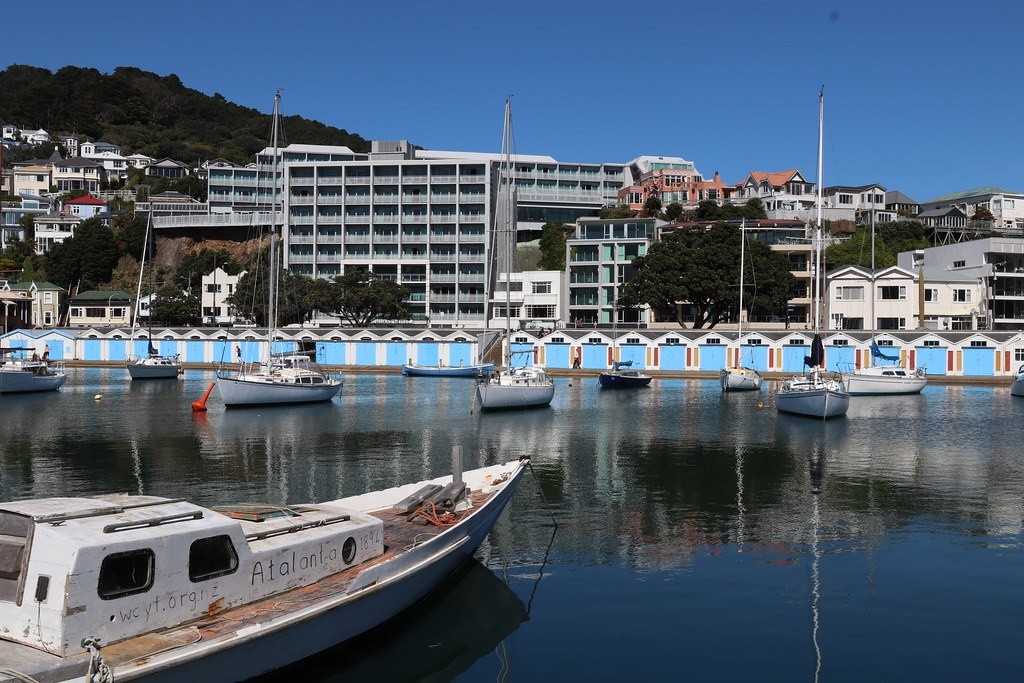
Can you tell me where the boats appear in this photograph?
[597,321,652,389]
[1010,364,1024,399]
[0,443,532,683]
[403,362,494,378]
[0,346,68,395]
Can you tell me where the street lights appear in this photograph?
[108,292,118,326]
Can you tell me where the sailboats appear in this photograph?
[123,199,187,380]
[836,186,930,395]
[774,84,850,421]
[472,94,556,414]
[210,86,346,411]
[721,217,763,391]
